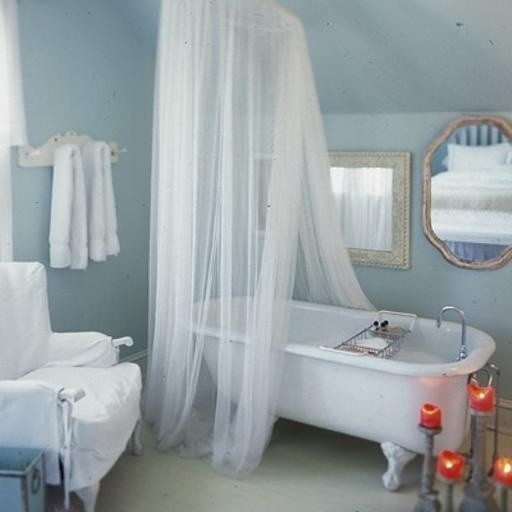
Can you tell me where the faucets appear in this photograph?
[438,305,468,361]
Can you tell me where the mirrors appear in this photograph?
[327,152,412,271]
[421,113,512,271]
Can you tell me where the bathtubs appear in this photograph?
[183,295,496,459]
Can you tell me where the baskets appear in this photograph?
[321,310,418,360]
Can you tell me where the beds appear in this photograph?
[431,171,512,263]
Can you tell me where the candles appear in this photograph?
[420,379,512,485]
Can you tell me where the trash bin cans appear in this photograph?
[0,447,45,512]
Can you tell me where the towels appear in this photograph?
[50,140,121,272]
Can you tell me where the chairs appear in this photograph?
[0,263,142,512]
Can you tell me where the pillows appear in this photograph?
[445,139,508,175]
